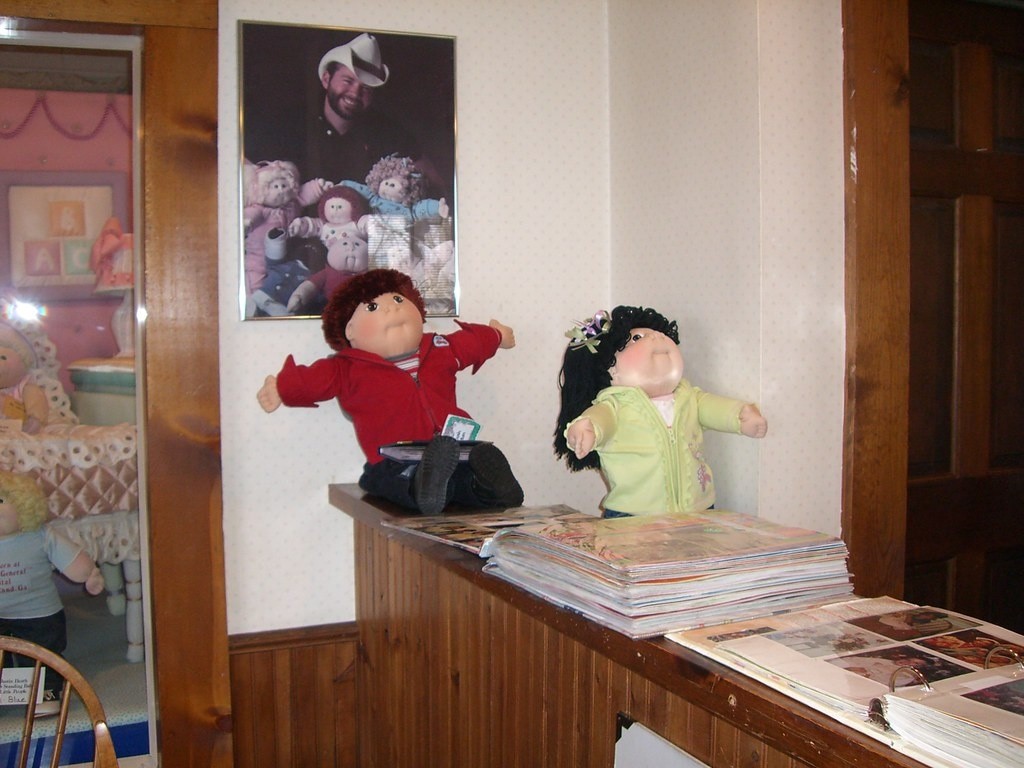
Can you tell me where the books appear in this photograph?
[379,500,1024,768]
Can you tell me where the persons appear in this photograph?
[552,305,768,517]
[294,32,448,199]
[0,331,52,435]
[256,269,525,516]
[0,471,105,721]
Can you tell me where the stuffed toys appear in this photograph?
[244,157,454,317]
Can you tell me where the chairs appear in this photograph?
[0,636,118,768]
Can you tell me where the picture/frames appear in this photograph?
[235,19,461,323]
[0,169,129,304]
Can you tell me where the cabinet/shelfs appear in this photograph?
[328,483,928,768]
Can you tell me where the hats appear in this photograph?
[317,33,390,87]
